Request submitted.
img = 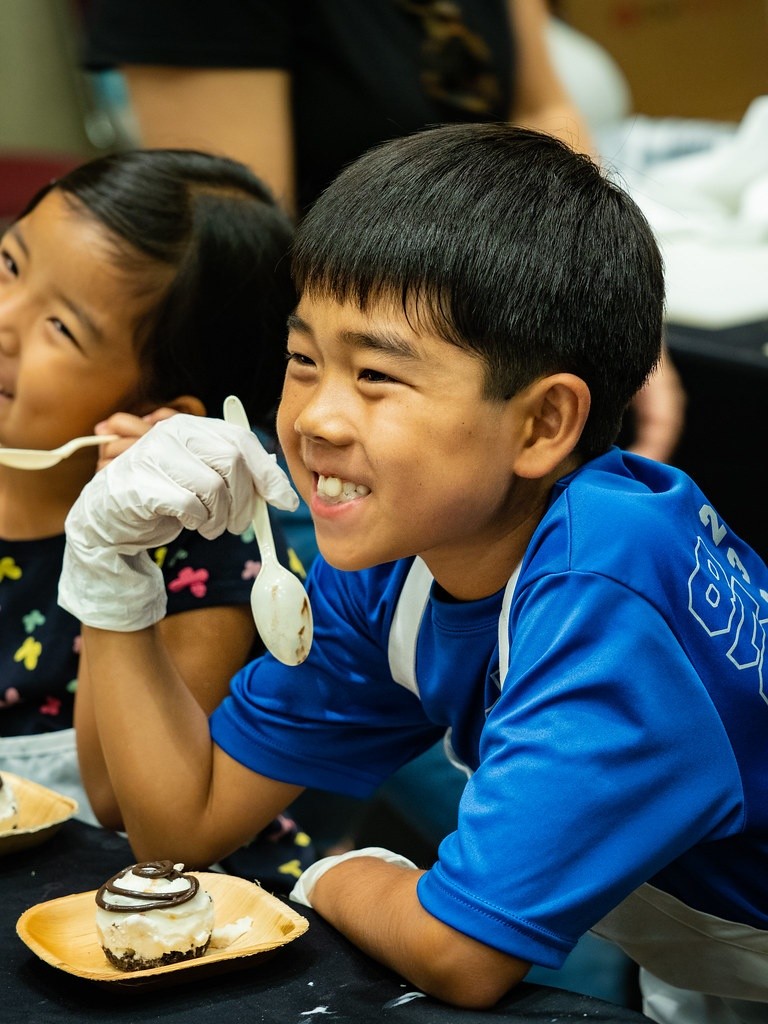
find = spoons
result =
[0,434,119,471]
[223,396,314,667]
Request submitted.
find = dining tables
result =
[0,819,658,1024]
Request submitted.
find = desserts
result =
[0,774,19,832]
[94,858,214,972]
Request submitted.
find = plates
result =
[0,770,79,851]
[16,872,311,981]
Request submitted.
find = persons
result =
[62,1,685,459]
[1,148,297,831]
[58,126,768,1006]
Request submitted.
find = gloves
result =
[287,847,419,908]
[58,413,304,632]
